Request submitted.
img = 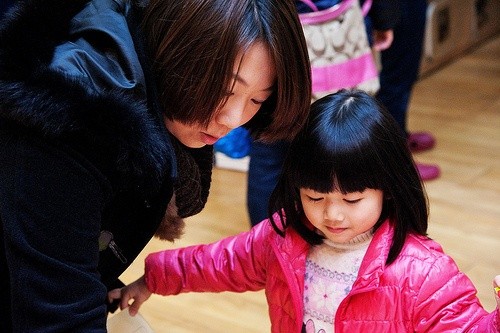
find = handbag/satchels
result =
[298,2,380,101]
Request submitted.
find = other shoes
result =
[405,131,435,150]
[414,160,439,181]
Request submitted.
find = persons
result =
[246,0,394,227]
[375,0,441,182]
[108,87,500,333]
[0,1,312,332]
